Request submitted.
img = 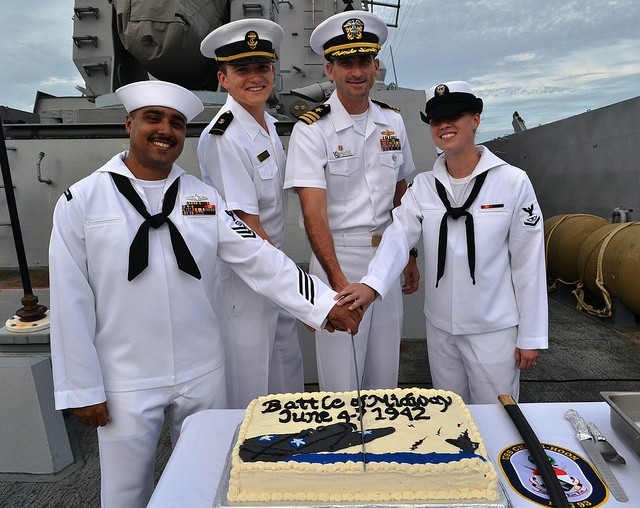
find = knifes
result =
[347,325,366,472]
[563,408,631,503]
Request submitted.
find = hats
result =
[114,80,204,124]
[199,18,285,66]
[420,80,483,125]
[309,9,388,59]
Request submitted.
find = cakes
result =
[225,386,502,503]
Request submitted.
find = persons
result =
[47,80,362,507]
[333,82,550,403]
[198,18,306,409]
[281,10,420,390]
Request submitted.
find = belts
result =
[334,235,383,247]
